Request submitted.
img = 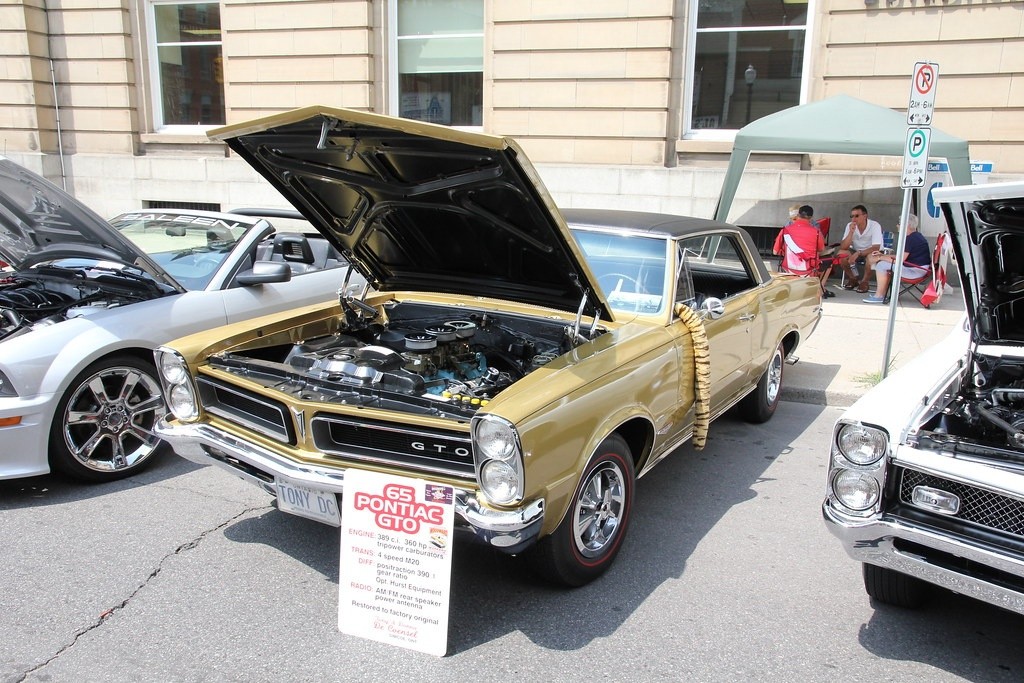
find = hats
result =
[799,205,813,219]
[787,204,801,219]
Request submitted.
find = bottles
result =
[849,263,858,277]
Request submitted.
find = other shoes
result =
[823,290,835,299]
[856,282,869,293]
[845,278,859,289]
[863,295,890,303]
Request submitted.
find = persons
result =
[862,213,932,303]
[840,205,885,294]
[785,205,835,298]
[773,205,824,278]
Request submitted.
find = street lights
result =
[744,65,756,125]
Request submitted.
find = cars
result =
[823,181,1024,618]
[151,105,824,590]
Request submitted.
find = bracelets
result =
[859,250,862,254]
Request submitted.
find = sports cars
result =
[0,155,376,483]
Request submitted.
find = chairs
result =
[883,234,944,309]
[817,218,830,246]
[206,228,322,277]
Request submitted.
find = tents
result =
[698,92,972,265]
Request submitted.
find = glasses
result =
[850,214,865,218]
